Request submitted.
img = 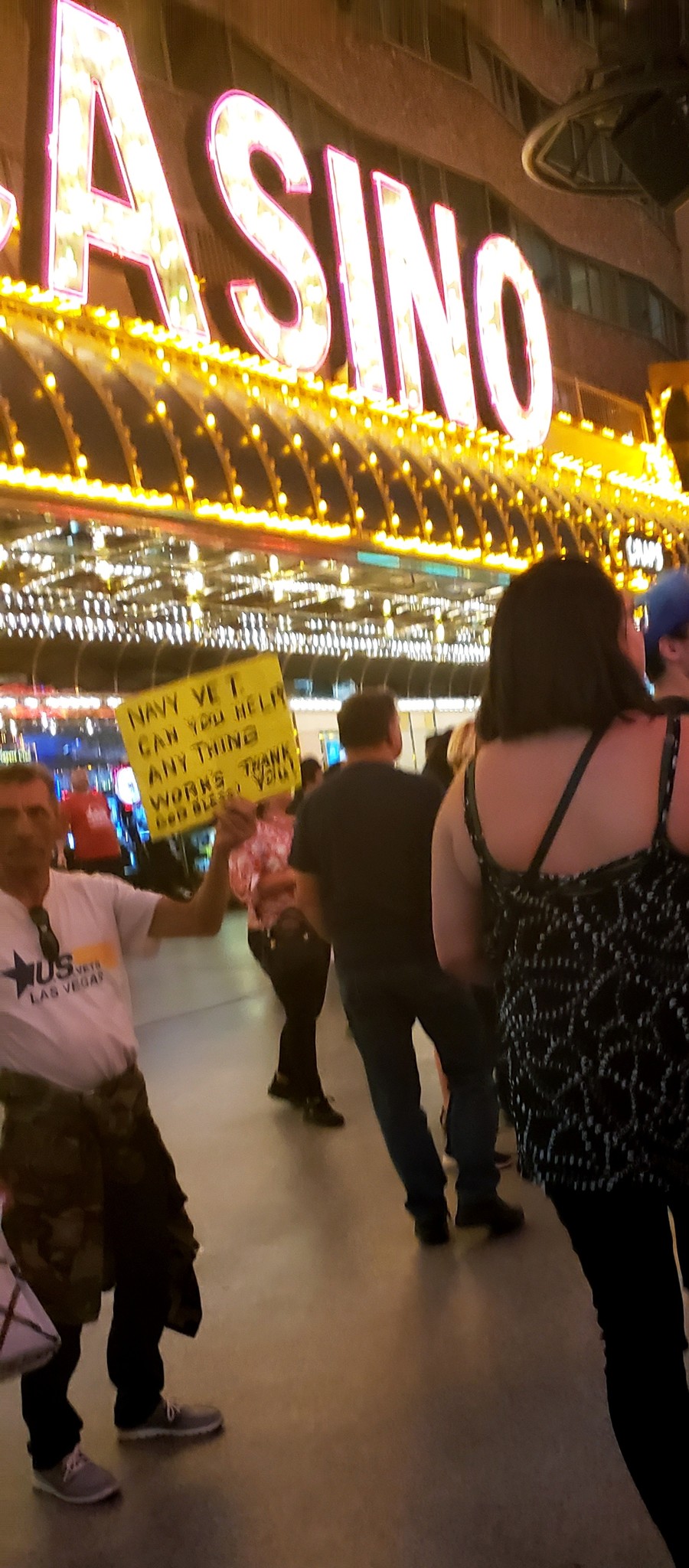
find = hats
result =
[631,575,689,641]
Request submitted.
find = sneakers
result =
[116,1396,222,1439]
[32,1448,118,1505]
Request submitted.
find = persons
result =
[54,765,127,879]
[430,558,689,1568]
[0,765,256,1504]
[286,692,522,1243]
[228,758,346,1126]
[643,566,689,700]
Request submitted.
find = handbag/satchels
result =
[249,849,318,961]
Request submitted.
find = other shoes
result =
[413,1217,449,1246]
[267,1073,300,1102]
[457,1197,525,1228]
[305,1101,347,1127]
